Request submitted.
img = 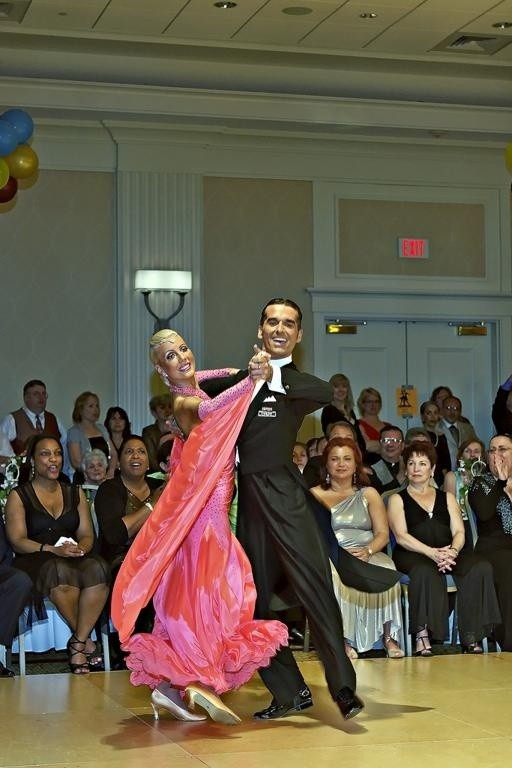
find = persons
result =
[165,298,365,724]
[110,329,290,724]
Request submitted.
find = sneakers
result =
[0,660,16,678]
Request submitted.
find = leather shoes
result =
[252,686,314,723]
[331,690,365,722]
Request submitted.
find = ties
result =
[33,415,44,434]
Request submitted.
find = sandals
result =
[66,632,104,675]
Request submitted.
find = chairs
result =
[14,483,111,677]
[380,487,488,658]
[464,491,504,653]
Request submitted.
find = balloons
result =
[0,108,39,215]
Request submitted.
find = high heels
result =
[381,634,405,659]
[460,640,483,655]
[413,634,434,656]
[345,647,359,659]
[148,687,207,722]
[184,681,242,727]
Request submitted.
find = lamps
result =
[133,269,192,331]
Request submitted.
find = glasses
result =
[442,404,458,414]
[378,437,401,446]
[486,445,509,456]
[462,446,483,457]
